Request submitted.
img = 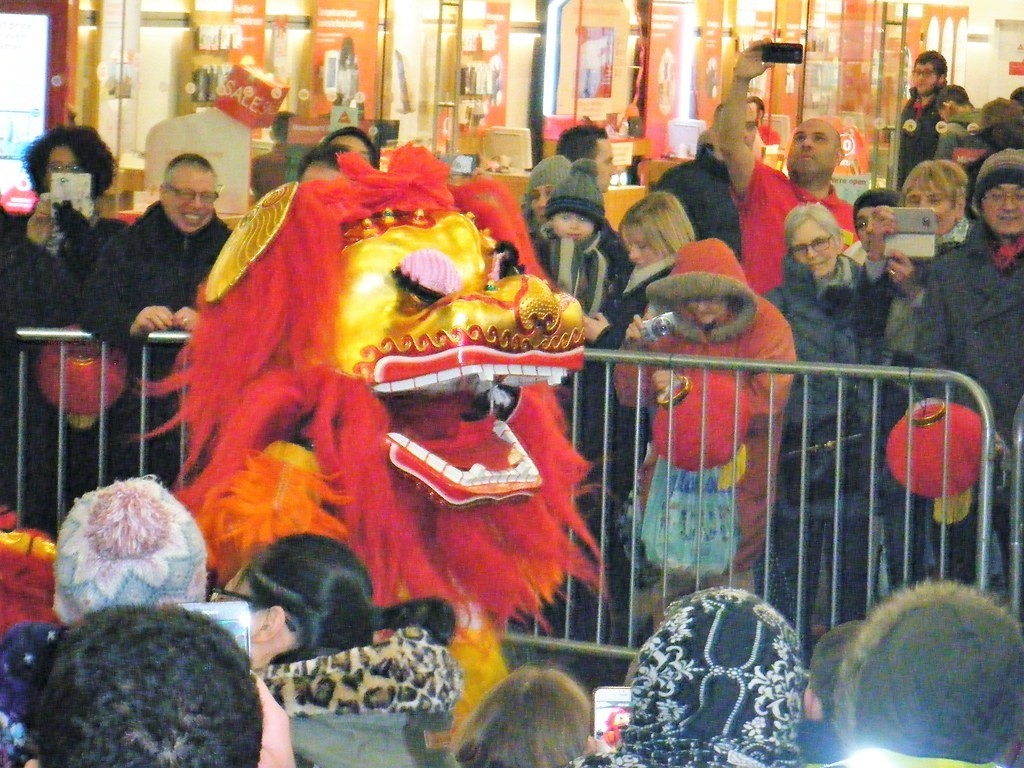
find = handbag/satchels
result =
[641,451,738,578]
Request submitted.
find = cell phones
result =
[888,207,935,234]
[49,173,92,219]
[181,600,252,659]
[590,686,634,755]
[762,42,803,64]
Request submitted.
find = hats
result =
[974,149,1024,203]
[51,481,209,628]
[546,156,606,224]
[853,187,900,218]
[526,154,573,190]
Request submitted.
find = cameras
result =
[640,312,677,344]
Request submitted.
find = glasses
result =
[165,185,220,204]
[854,218,870,232]
[48,163,85,174]
[789,235,836,257]
[203,583,296,640]
[910,68,938,78]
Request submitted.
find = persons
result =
[0,35,1023,768]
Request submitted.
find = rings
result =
[887,269,896,277]
[181,317,189,325]
